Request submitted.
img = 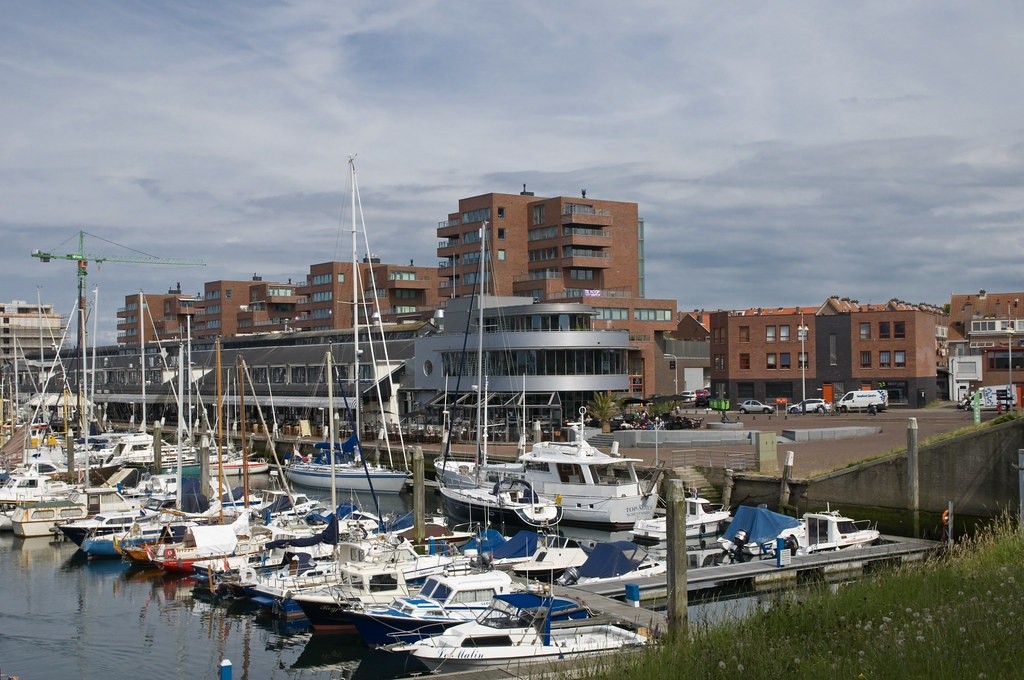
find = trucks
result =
[957,384,1018,412]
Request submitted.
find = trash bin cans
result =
[709,398,730,410]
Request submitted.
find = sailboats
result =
[1,156,881,676]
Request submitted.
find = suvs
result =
[787,398,830,415]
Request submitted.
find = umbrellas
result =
[399,411,432,428]
[619,397,649,414]
[364,409,392,424]
[653,395,687,401]
[25,395,95,407]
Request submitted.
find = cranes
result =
[30,231,207,359]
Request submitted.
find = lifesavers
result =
[240,568,257,583]
[163,548,176,559]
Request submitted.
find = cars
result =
[695,389,710,398]
[738,399,776,415]
[679,390,698,403]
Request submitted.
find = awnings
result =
[91,394,363,410]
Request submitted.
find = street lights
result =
[443,411,452,452]
[213,403,218,435]
[798,312,809,415]
[1003,314,1016,413]
[129,401,134,429]
[318,407,325,433]
[655,417,659,467]
[663,354,678,394]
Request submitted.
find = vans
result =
[835,389,889,413]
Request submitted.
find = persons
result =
[634,409,648,420]
